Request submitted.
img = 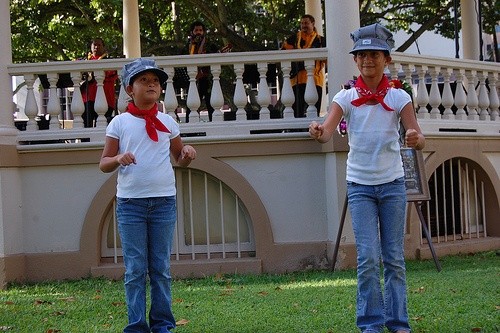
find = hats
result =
[122,58,168,89]
[349,23,395,54]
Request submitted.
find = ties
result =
[127,101,171,142]
[351,75,395,111]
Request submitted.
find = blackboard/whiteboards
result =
[400,149,423,196]
